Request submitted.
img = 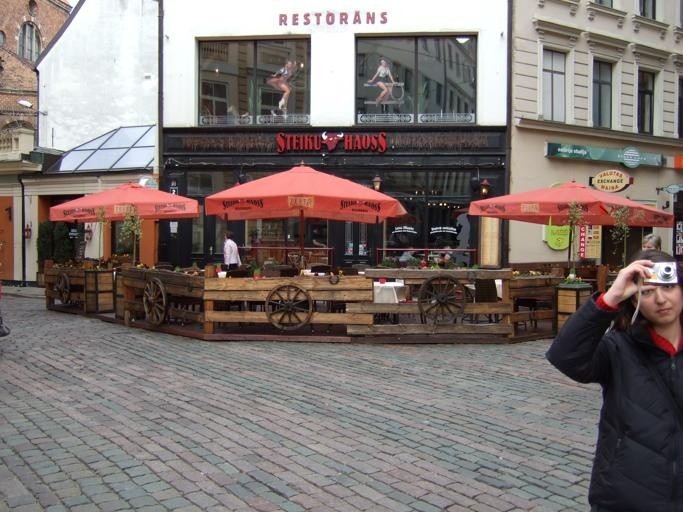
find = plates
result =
[302,270,325,277]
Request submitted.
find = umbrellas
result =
[468,179,674,270]
[48,181,198,266]
[204,160,406,269]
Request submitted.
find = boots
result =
[279,98,285,110]
[375,97,383,109]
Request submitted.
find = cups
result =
[218,271,226,278]
[357,272,365,276]
[379,279,386,284]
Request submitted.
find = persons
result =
[640,232,663,254]
[222,228,243,278]
[398,247,417,261]
[545,249,683,511]
[366,58,398,111]
[308,229,326,263]
[267,59,297,115]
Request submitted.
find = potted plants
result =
[569,268,577,281]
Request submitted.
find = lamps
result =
[17,100,47,116]
[84,224,92,240]
[371,174,383,190]
[24,224,32,239]
[479,179,491,199]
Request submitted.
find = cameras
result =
[642,261,678,285]
